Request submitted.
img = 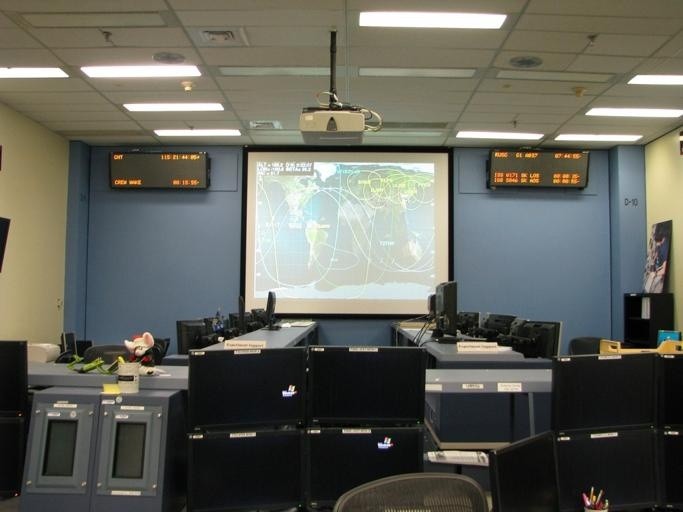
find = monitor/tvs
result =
[307,426,422,512]
[205,318,239,342]
[186,430,307,511]
[490,431,558,512]
[84,344,132,362]
[659,429,682,511]
[512,320,562,358]
[266,291,280,333]
[0,415,30,503]
[457,311,479,337]
[477,312,517,342]
[431,281,459,344]
[556,429,662,511]
[552,353,658,433]
[657,354,683,433]
[251,308,267,329]
[310,345,426,428]
[242,312,259,334]
[229,313,245,336]
[0,340,28,416]
[176,319,218,355]
[189,348,309,432]
[60,332,78,357]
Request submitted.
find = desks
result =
[203,320,317,350]
[388,320,525,365]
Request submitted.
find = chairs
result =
[568,337,612,357]
[334,471,488,511]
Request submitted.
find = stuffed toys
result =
[124,332,157,376]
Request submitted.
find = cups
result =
[583,506,609,512]
[118,363,140,393]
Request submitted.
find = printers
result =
[28,343,61,364]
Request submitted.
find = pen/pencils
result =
[581,486,609,510]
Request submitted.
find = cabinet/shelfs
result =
[623,292,674,348]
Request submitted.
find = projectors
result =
[299,105,364,146]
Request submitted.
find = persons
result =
[640,223,670,293]
